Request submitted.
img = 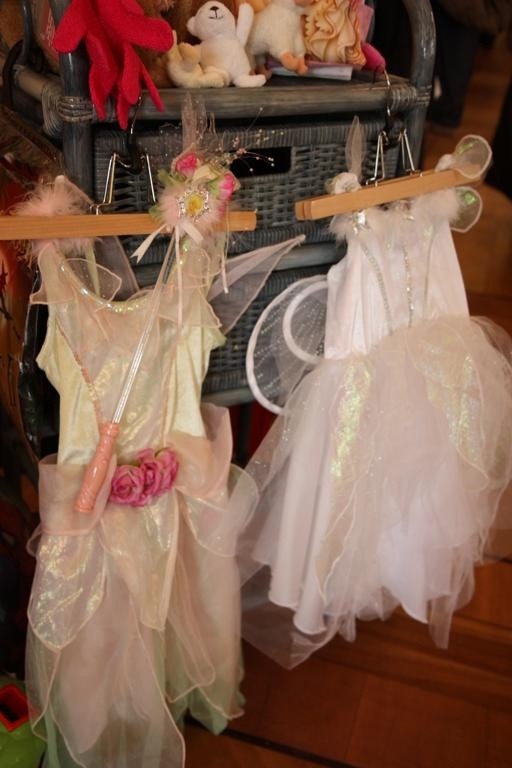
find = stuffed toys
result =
[242,1,308,80]
[161,31,231,90]
[185,1,265,89]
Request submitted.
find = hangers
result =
[1,95,257,243]
[294,63,481,222]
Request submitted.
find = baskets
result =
[95,120,403,267]
[134,243,347,392]
